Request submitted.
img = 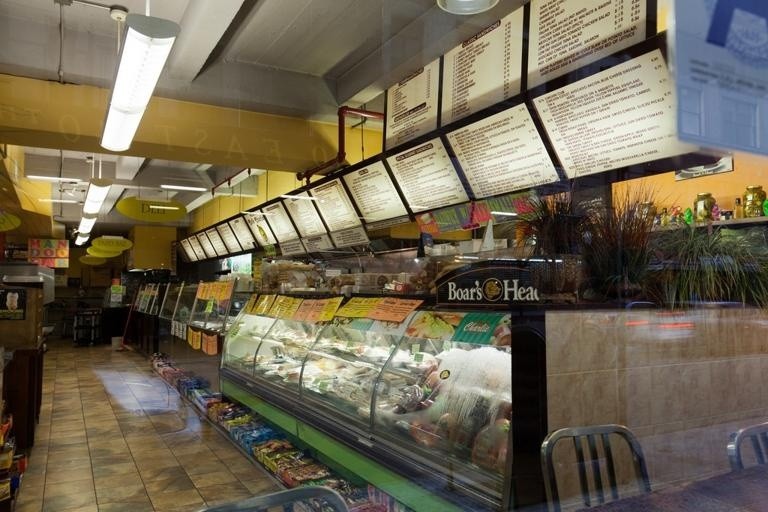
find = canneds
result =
[639,202,658,226]
[693,192,717,221]
[719,209,733,221]
[742,185,766,216]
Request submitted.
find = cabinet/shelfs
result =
[0,275,46,485]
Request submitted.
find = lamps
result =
[57,0,181,246]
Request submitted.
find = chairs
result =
[539,422,766,511]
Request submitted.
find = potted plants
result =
[500,172,768,310]
